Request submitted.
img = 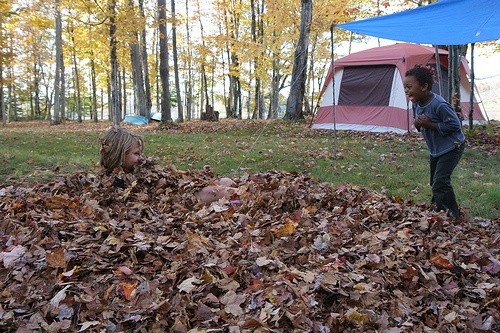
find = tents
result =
[305,42,491,136]
[331,0,500,229]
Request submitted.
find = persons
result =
[403,66,468,221]
[97,125,249,203]
